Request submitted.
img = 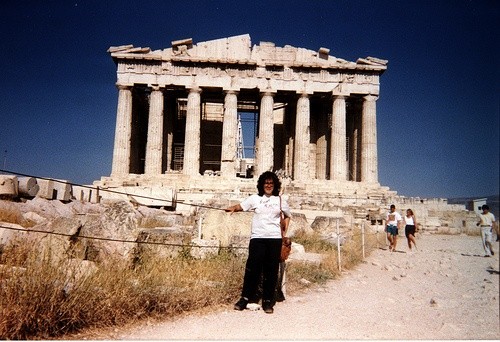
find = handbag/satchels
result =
[280,237,292,263]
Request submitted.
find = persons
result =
[385,205,403,252]
[401,209,419,252]
[225,171,291,314]
[476,205,496,257]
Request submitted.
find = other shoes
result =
[262,301,273,314]
[235,297,249,310]
